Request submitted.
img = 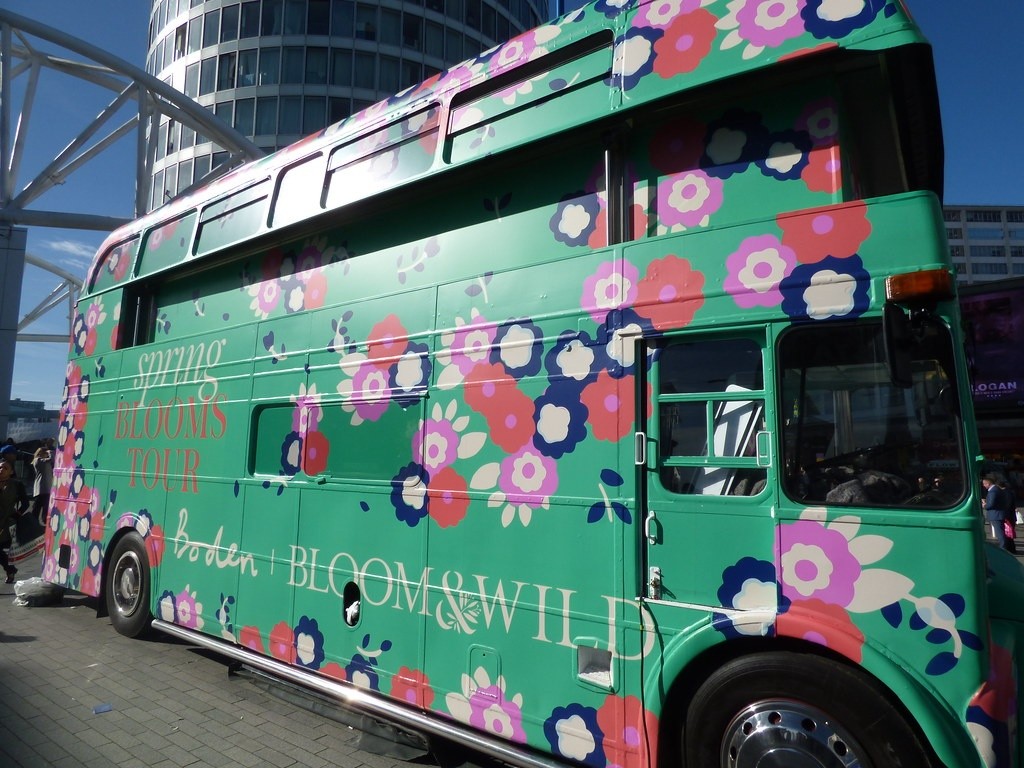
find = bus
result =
[42,0,1023,768]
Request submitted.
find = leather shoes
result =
[5,569,17,582]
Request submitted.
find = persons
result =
[982,477,1011,549]
[997,479,1017,553]
[0,459,29,584]
[2,438,17,479]
[29,447,53,525]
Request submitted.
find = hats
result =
[0,445,21,455]
